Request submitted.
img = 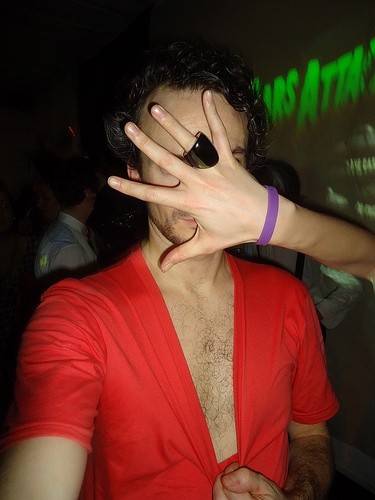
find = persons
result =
[0,41,341,500]
[238,160,364,345]
[107,90,375,285]
[34,157,100,294]
[0,172,72,400]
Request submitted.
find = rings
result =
[183,131,219,169]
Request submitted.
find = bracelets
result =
[256,184,279,246]
